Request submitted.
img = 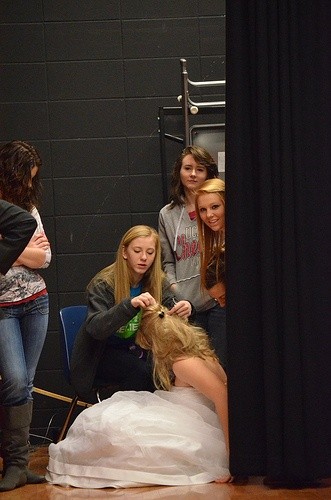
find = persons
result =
[157,144,225,373]
[68,224,196,404]
[0,139,52,492]
[203,250,225,310]
[195,179,225,283]
[0,198,38,276]
[47,304,229,489]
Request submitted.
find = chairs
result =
[58,305,89,442]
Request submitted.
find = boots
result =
[25,400,46,483]
[0,401,33,493]
